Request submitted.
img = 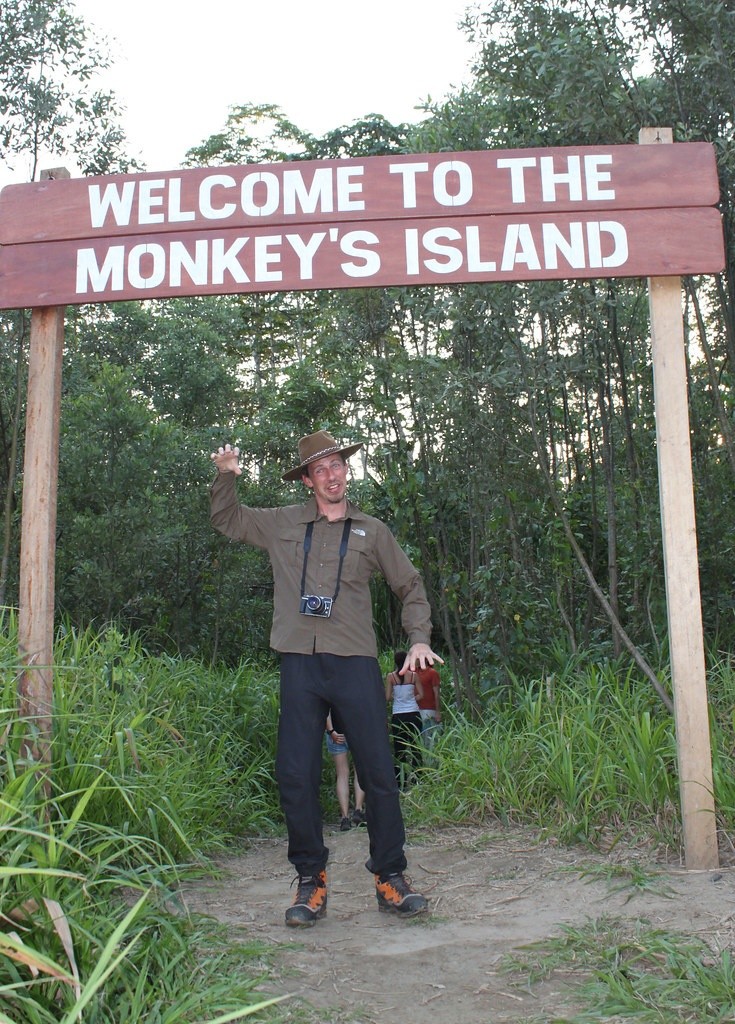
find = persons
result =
[208,429,445,926]
[325,651,441,830]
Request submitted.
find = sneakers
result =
[340,818,352,831]
[284,869,328,926]
[353,809,366,825]
[374,873,429,919]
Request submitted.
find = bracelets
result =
[327,729,335,735]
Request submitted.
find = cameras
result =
[300,595,333,619]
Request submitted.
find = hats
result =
[281,428,364,481]
